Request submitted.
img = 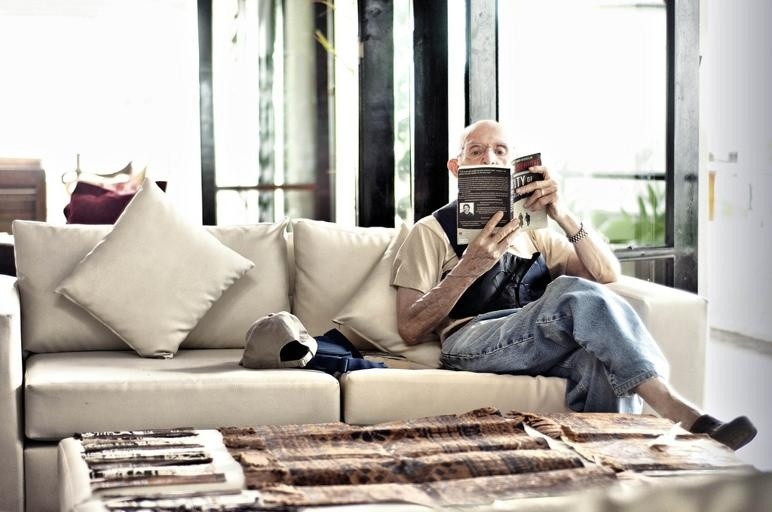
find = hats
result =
[241,311,317,368]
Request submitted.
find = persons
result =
[389,120,758,451]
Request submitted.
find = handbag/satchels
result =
[311,329,387,372]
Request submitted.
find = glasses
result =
[456,145,510,159]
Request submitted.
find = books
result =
[457,153,547,245]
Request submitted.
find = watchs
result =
[565,223,588,243]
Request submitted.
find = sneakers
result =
[690,414,756,451]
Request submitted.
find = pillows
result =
[64,180,168,226]
[330,218,444,370]
[290,215,401,354]
[11,216,294,360]
[53,176,257,361]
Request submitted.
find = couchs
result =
[0,218,708,512]
[56,412,745,511]
